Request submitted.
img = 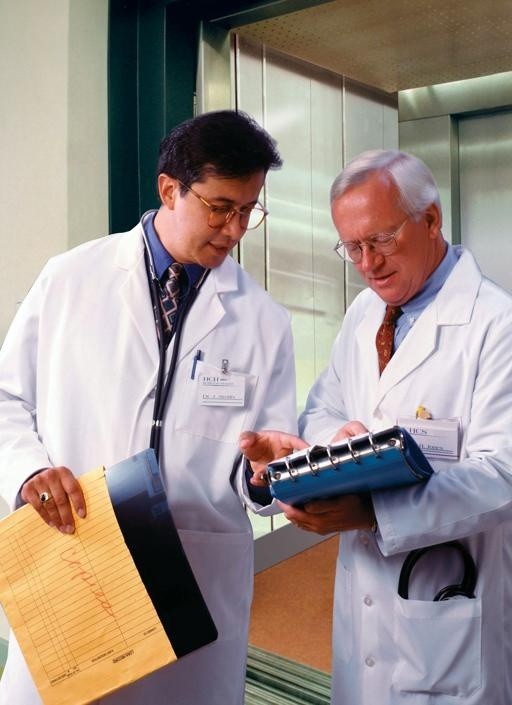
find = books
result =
[265,427,432,506]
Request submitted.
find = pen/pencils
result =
[190,350,202,381]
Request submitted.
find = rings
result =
[39,492,52,502]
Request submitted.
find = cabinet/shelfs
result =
[227,33,400,575]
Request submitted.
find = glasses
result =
[332,211,412,262]
[172,177,269,232]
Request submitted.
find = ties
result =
[153,262,183,354]
[375,304,404,377]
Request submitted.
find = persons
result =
[3,109,312,703]
[299,149,512,704]
